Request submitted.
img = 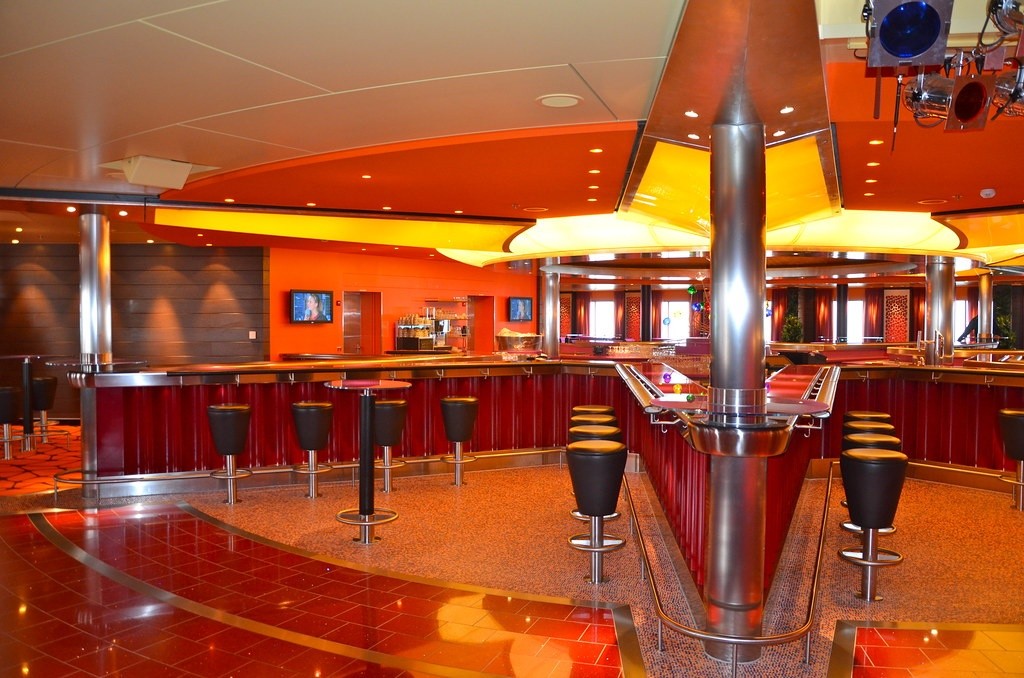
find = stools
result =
[30,377,57,443]
[292,401,334,498]
[441,397,478,487]
[209,404,251,504]
[373,400,407,492]
[997,409,1024,511]
[0,387,26,460]
[839,412,909,602]
[566,405,628,588]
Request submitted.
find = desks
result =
[0,354,71,451]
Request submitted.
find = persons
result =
[956,315,977,341]
[516,300,528,318]
[304,293,328,321]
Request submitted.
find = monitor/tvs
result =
[290,289,333,324]
[508,297,532,321]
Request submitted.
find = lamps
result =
[864,0,1024,131]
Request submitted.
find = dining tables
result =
[323,381,412,546]
